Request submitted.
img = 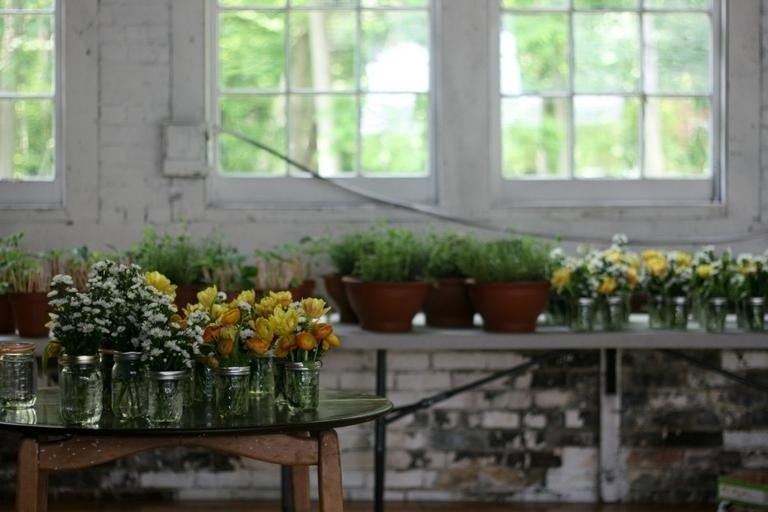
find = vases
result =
[0,342,39,424]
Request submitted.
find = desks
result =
[1,323,768,512]
[0,375,394,512]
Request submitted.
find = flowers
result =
[549,231,768,331]
